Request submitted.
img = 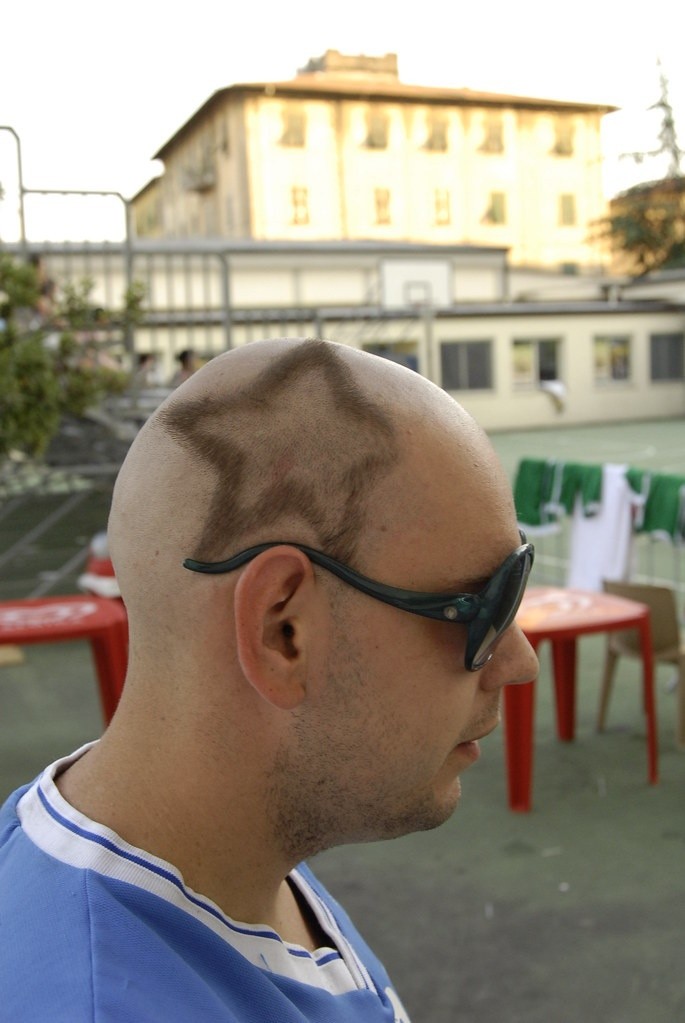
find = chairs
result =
[598,581,685,745]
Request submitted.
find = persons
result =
[0,337,539,1023]
[130,348,198,389]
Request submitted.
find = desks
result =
[480,584,661,814]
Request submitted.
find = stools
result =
[0,595,129,728]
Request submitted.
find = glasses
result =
[184,527,538,674]
